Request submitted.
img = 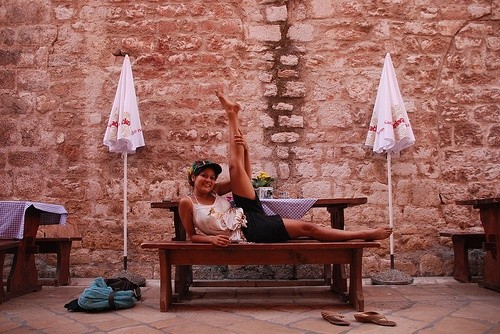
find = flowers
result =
[252,172,274,188]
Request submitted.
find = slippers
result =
[321,311,350,324]
[354,312,396,326]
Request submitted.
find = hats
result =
[190,160,222,175]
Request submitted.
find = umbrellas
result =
[103,53,146,271]
[366,51,415,270]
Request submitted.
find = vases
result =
[255,187,273,199]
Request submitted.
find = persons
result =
[178,88,393,247]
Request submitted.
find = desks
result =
[456,197,500,293]
[151,197,368,299]
[0,201,67,297]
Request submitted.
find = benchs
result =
[141,237,380,313]
[439,231,500,293]
[0,237,82,304]
